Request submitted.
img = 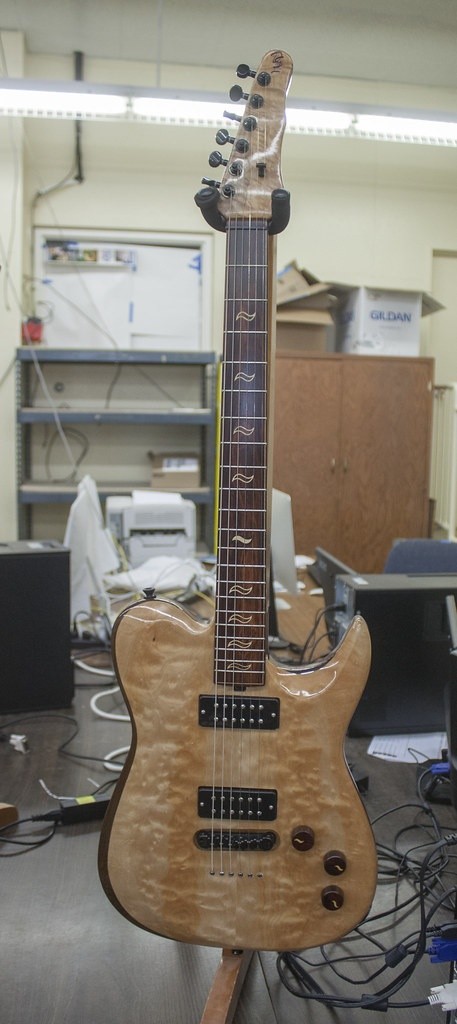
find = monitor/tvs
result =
[316,547,360,648]
[270,486,298,648]
[417,595,456,805]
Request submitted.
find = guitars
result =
[93,48,376,951]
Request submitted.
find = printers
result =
[105,497,197,571]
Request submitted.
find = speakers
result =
[0,539,75,711]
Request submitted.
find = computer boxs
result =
[333,572,457,737]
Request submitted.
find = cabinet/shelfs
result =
[273,350,435,573]
[18,345,218,565]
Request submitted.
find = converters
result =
[32,794,112,824]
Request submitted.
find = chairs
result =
[384,537,457,574]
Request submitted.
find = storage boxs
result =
[148,450,204,487]
[328,281,447,356]
[275,307,334,350]
